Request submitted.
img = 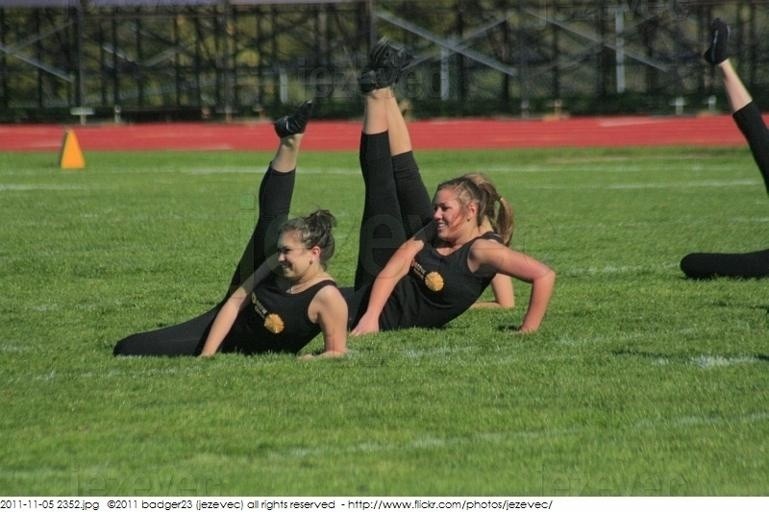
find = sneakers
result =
[275,97,316,139]
[357,36,402,92]
[703,17,731,65]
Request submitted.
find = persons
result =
[681,18,768,277]
[333,50,556,337]
[367,35,517,310]
[113,99,348,358]
